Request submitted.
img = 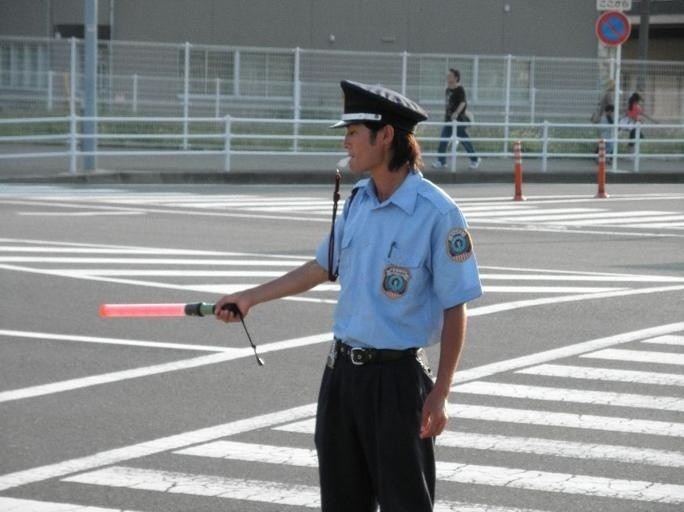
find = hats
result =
[328,80,427,133]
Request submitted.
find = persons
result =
[602,79,615,119]
[214,81,484,512]
[430,69,483,170]
[626,92,658,147]
[596,104,616,165]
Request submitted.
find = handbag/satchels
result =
[464,110,473,121]
[619,117,634,131]
[590,106,601,124]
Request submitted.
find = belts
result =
[334,341,418,362]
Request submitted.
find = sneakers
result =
[469,158,481,168]
[431,161,448,168]
